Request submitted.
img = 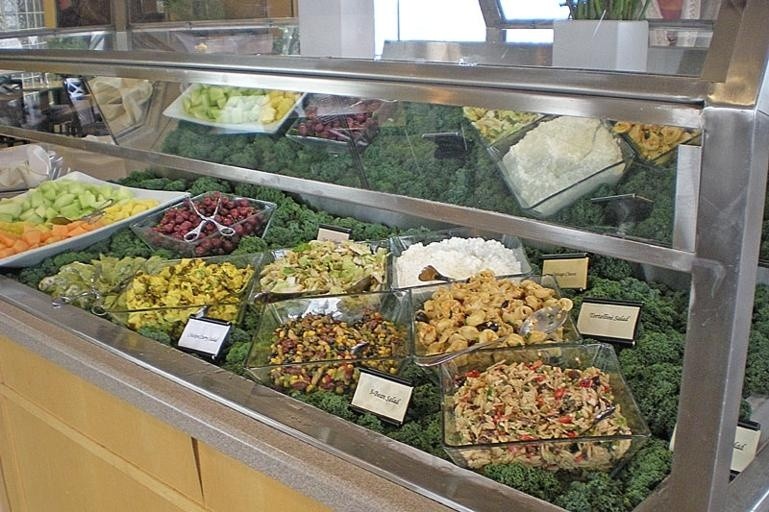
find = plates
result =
[1,171,193,268]
[164,83,312,133]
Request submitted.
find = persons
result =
[647,21,715,49]
[633,0,720,21]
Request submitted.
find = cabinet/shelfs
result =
[0,0,769,512]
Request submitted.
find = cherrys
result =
[146,192,268,257]
[299,98,369,142]
